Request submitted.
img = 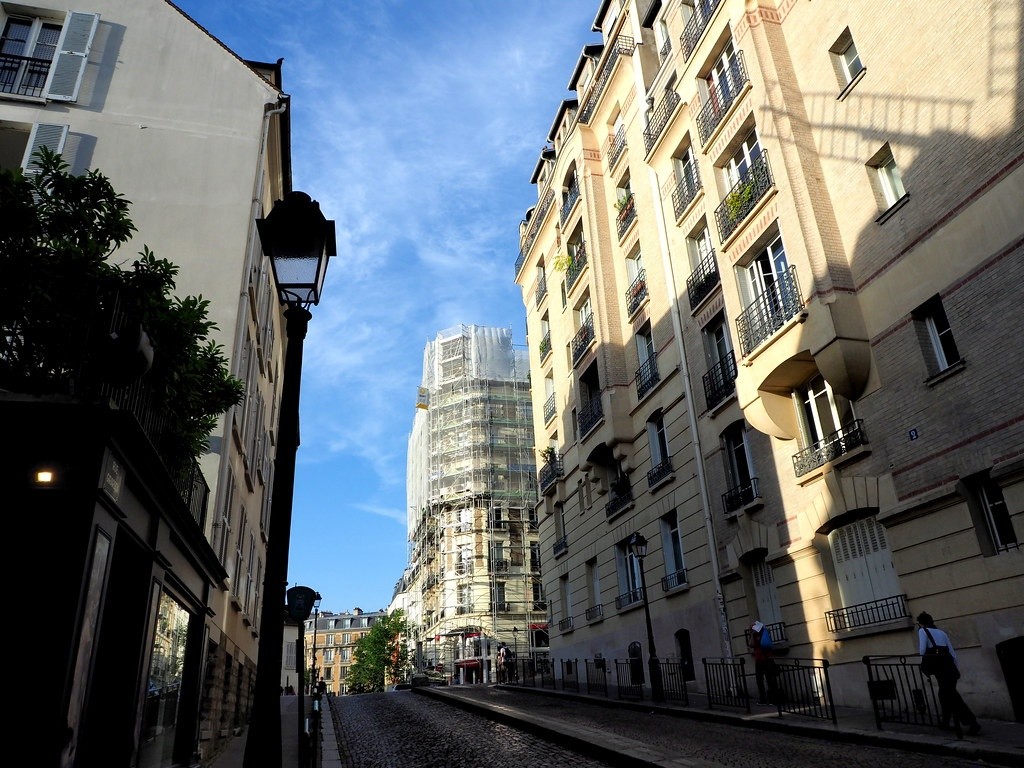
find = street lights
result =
[310,591,323,696]
[511,626,518,678]
[629,531,666,704]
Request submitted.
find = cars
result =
[391,682,412,692]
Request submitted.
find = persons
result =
[318,676,327,693]
[748,622,778,707]
[287,685,296,695]
[916,611,982,736]
[496,642,518,683]
[393,673,401,691]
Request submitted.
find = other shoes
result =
[756,701,767,706]
[769,701,773,705]
[939,723,950,733]
[965,723,981,736]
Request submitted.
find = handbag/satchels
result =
[921,628,950,673]
[761,628,774,654]
